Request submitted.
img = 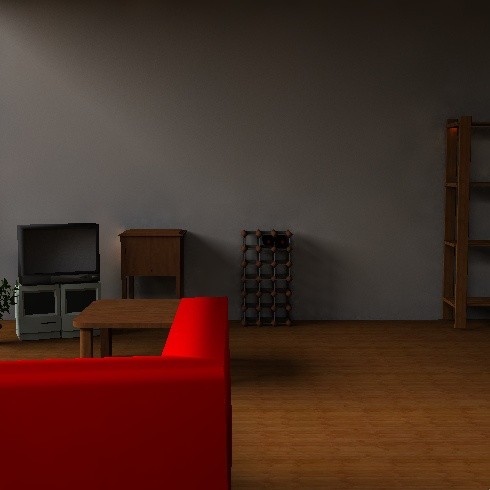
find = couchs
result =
[0,296,231,490]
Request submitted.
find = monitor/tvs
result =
[17,222,101,285]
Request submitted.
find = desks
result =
[118,229,187,298]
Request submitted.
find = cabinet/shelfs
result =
[15,283,101,341]
[239,229,292,326]
[442,115,490,329]
